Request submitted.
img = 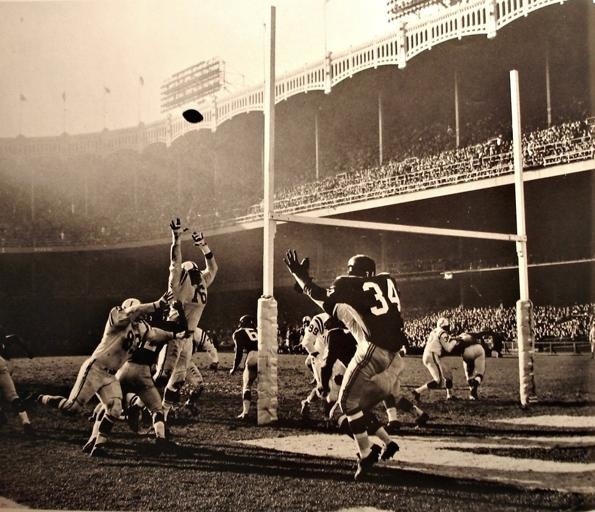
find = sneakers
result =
[411,390,420,401]
[24,424,33,434]
[16,392,39,412]
[157,445,182,457]
[354,444,381,481]
[83,409,125,458]
[128,405,201,432]
[382,442,398,460]
[447,396,460,402]
[468,379,478,399]
[294,257,309,294]
[299,400,428,438]
[236,414,248,420]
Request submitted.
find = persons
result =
[411,317,486,399]
[206,246,595,353]
[1,356,34,432]
[284,248,404,479]
[301,311,428,431]
[127,218,218,435]
[0,90,594,245]
[21,291,188,456]
[230,315,258,418]
[81,301,219,451]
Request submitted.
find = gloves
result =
[174,330,194,339]
[192,231,208,251]
[283,249,307,280]
[170,218,188,244]
[154,291,174,309]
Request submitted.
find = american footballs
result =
[182,109,203,123]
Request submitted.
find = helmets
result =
[346,255,375,277]
[121,298,163,321]
[238,314,257,328]
[437,318,450,331]
[181,260,200,284]
